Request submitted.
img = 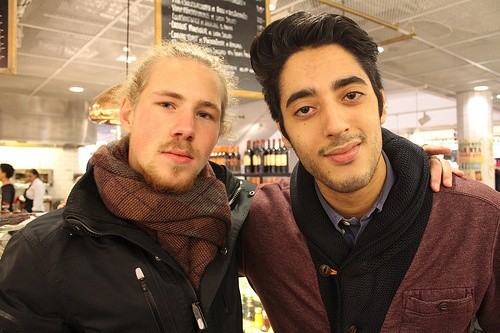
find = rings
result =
[427,155,440,161]
[420,144,428,147]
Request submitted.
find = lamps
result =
[88,0,131,125]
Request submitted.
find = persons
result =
[0,40,465,333]
[231,11,500,333]
[0,163,15,213]
[23,169,45,213]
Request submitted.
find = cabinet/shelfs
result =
[229,172,294,333]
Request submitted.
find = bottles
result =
[276,139,281,174]
[252,140,260,173]
[209,145,241,173]
[266,140,271,173]
[241,294,270,332]
[281,147,288,174]
[245,141,252,173]
[260,139,266,173]
[253,141,255,150]
[271,139,276,173]
